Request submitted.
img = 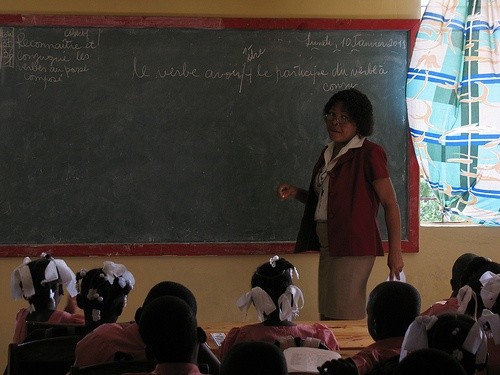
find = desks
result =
[196,311,375,362]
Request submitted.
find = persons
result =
[278,88,404,321]
[4,254,500,375]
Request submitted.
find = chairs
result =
[7,333,84,375]
[23,319,85,335]
[70,357,210,375]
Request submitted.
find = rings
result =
[399,269,402,272]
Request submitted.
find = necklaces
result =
[317,168,331,186]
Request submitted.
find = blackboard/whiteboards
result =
[0,12,421,257]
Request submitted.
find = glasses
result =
[325,113,352,123]
